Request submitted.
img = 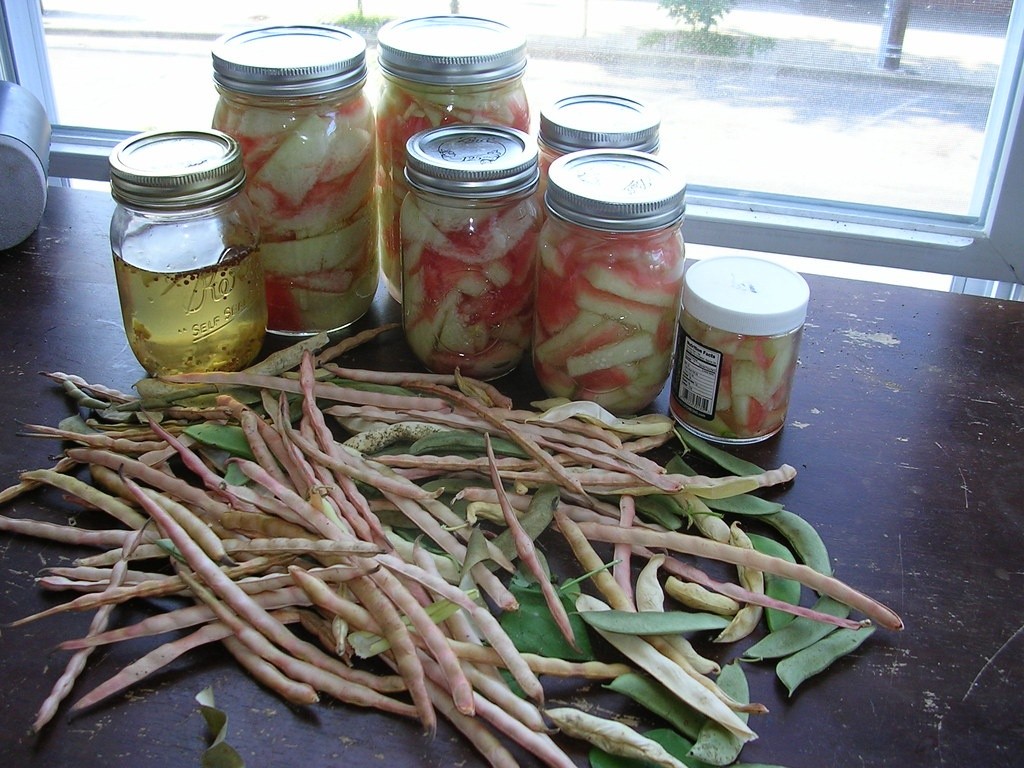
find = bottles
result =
[108,128,268,378]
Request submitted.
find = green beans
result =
[0,319,904,768]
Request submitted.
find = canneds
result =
[671,256,811,443]
